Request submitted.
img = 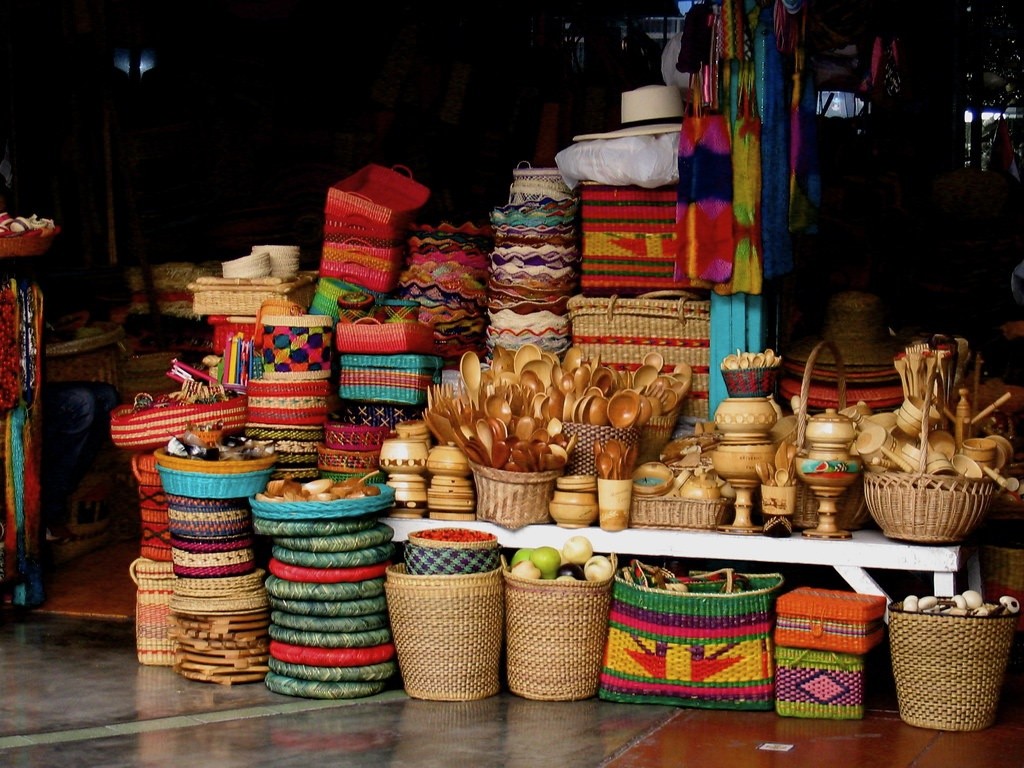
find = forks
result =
[427,384,465,457]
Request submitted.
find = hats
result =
[573,83,685,142]
[779,293,907,407]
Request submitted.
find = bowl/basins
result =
[549,491,598,528]
[714,398,777,437]
[0,213,33,234]
[302,479,332,501]
[380,439,428,477]
[427,442,471,480]
[806,409,854,448]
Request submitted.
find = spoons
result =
[595,439,632,480]
[544,386,652,429]
[755,442,797,487]
[466,394,578,473]
[592,352,692,418]
[460,351,481,411]
[721,349,782,370]
[492,344,592,394]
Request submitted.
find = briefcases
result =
[774,586,887,721]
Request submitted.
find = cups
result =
[598,477,632,531]
[761,485,796,515]
[855,396,1013,479]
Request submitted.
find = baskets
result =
[864,334,997,544]
[792,342,873,531]
[0,167,784,710]
[888,596,1018,732]
[136,664,605,768]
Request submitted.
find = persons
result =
[37,379,123,543]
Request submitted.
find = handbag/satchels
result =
[597,561,784,711]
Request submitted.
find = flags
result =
[991,118,1020,182]
